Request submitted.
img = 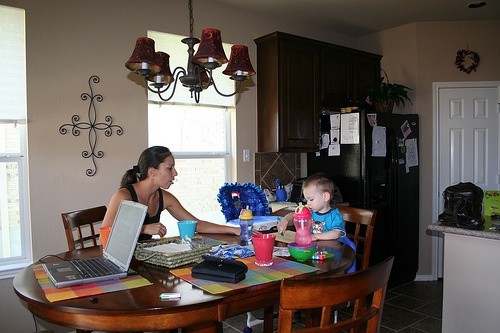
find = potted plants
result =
[359,77,413,114]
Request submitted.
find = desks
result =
[14,232,353,333]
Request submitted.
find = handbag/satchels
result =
[191,255,248,284]
[437,182,486,231]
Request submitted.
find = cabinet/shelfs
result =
[252,30,418,290]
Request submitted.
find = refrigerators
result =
[312,113,420,284]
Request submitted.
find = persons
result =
[99,146,262,241]
[277,173,357,273]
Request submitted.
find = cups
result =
[177,220,198,240]
[99,226,112,249]
[251,234,276,266]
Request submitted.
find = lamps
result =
[125,0,255,103]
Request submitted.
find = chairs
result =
[62,206,106,251]
[334,205,378,270]
[278,256,396,333]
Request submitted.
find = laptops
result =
[42,200,148,288]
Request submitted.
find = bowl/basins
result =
[287,241,318,262]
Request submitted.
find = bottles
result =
[239,205,254,242]
[293,202,313,246]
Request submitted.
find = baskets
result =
[133,238,212,268]
